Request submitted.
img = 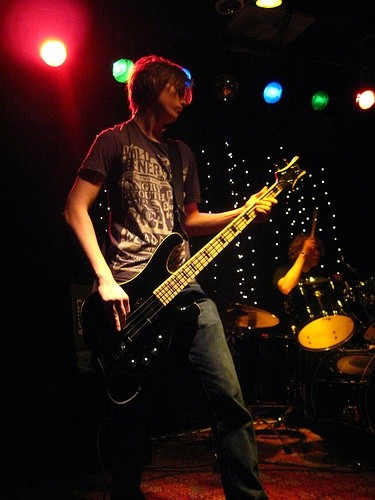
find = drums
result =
[310,349,375,454]
[351,278,375,342]
[284,277,355,351]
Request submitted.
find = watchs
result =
[301,252,308,256]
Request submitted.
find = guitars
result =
[79,156,307,404]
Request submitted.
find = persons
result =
[272,232,342,325]
[62,54,280,500]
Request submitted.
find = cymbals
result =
[222,304,279,328]
